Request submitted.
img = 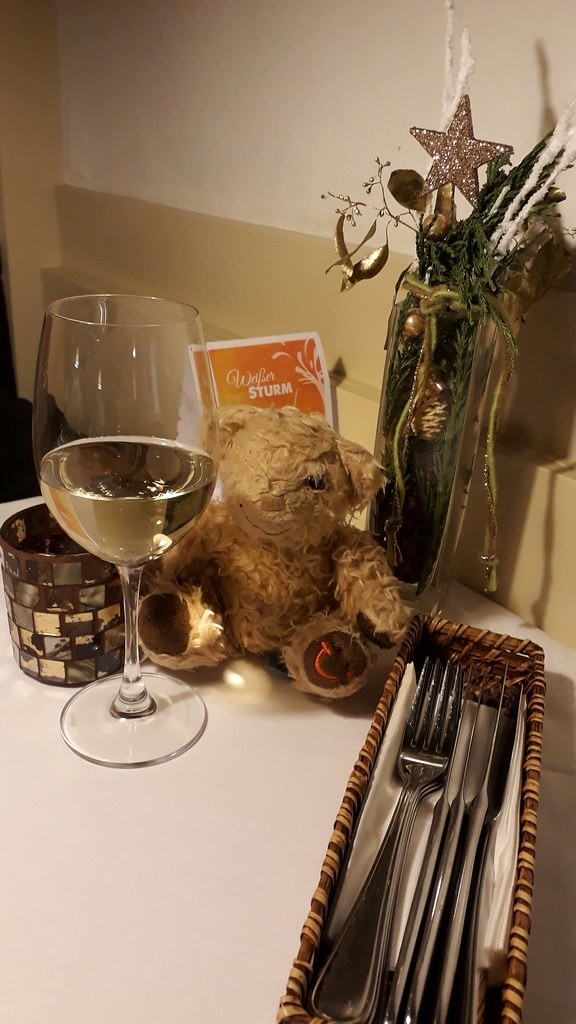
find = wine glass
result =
[32,291,224,769]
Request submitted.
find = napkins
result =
[323,662,526,990]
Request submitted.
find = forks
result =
[313,654,464,1024]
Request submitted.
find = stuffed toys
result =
[137,406,406,697]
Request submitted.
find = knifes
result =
[388,660,511,1024]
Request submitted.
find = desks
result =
[0,462,576,1024]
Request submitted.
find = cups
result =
[0,502,147,688]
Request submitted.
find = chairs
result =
[0,394,50,506]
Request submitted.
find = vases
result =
[364,271,504,603]
